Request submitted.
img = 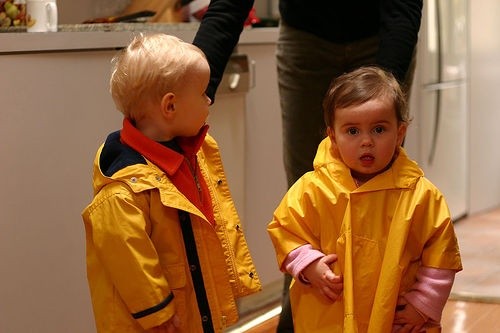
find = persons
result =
[266,67,463,333]
[193,0,423,333]
[81,35,262,333]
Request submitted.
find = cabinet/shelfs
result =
[0,26,286,333]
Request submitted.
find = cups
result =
[26,0,58,32]
